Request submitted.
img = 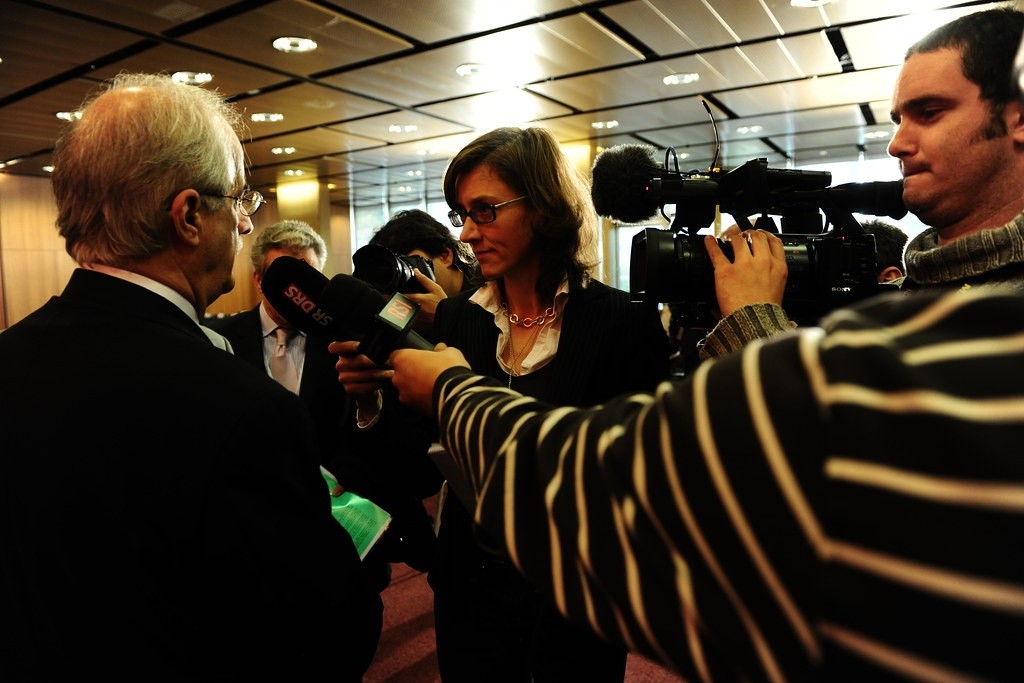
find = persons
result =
[205,7,1024,683]
[0,76,383,683]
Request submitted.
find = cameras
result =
[353,245,436,296]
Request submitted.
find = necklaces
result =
[499,296,554,328]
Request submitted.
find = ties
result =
[272,328,298,394]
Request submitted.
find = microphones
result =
[592,147,831,226]
[261,255,437,371]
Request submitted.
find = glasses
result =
[165,189,267,216]
[448,196,528,227]
